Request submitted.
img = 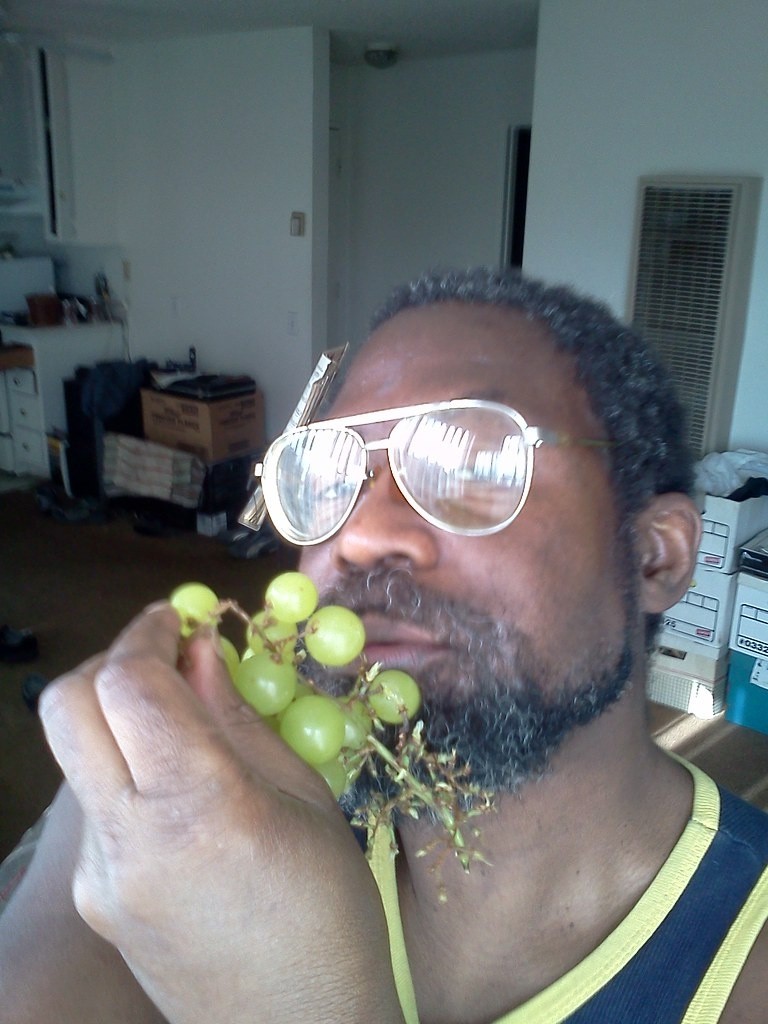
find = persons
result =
[0,268,768,1024]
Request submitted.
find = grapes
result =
[168,573,420,802]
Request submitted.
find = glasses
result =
[248,397,622,546]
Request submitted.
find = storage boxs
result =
[142,388,265,466]
[648,494,768,737]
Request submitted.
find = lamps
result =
[364,44,397,68]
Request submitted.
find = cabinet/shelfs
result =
[0,34,76,241]
[0,321,125,480]
